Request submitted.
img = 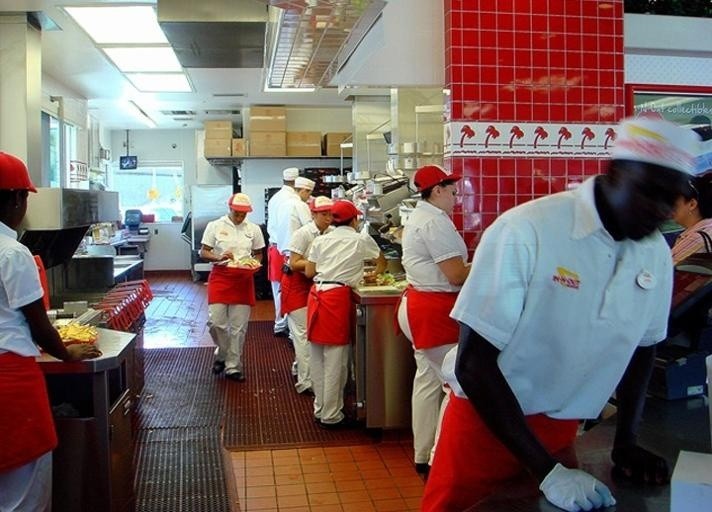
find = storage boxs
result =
[202,107,353,158]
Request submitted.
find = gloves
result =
[539,461,617,512]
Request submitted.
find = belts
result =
[314,281,346,286]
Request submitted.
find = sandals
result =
[212,359,224,374]
[225,372,246,383]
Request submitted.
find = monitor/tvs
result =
[120,156,137,169]
[125,209,143,230]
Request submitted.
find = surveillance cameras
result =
[172,144,176,148]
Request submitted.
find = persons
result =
[0,151,102,512]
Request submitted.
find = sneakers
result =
[415,462,431,478]
[314,414,357,430]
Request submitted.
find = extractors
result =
[0,10,121,272]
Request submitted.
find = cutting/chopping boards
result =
[356,282,398,292]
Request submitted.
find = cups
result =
[325,171,383,200]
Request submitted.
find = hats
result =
[309,195,334,212]
[228,192,254,213]
[330,200,364,223]
[604,116,704,177]
[414,166,461,192]
[294,176,317,191]
[0,149,39,195]
[282,167,299,182]
